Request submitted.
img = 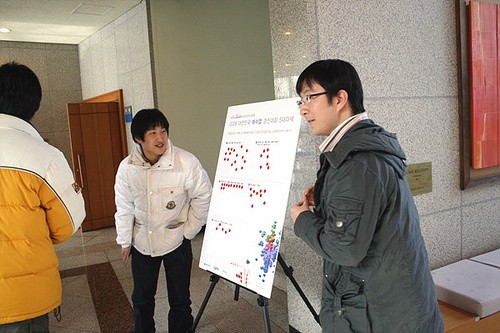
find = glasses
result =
[295,90,334,108]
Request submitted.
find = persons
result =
[1,61,86,332]
[289,60,445,333]
[115,108,213,333]
